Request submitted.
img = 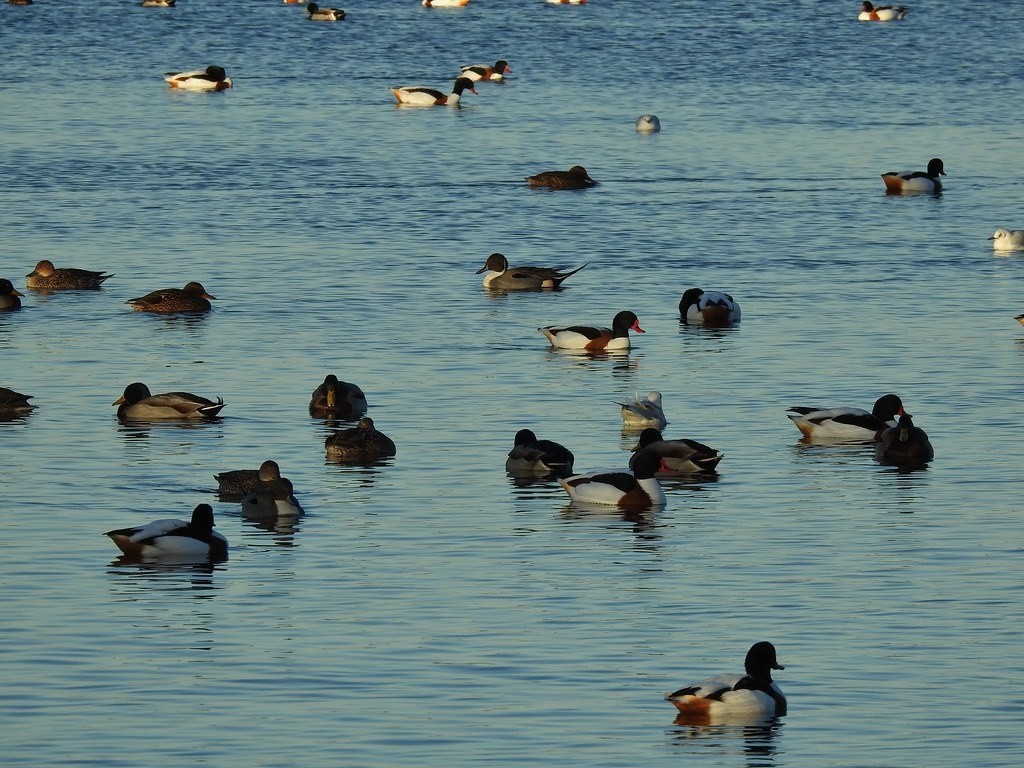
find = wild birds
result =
[505,310,726,510]
[283,0,588,21]
[662,642,789,719]
[457,60,512,81]
[142,0,233,92]
[0,259,396,568]
[523,165,598,192]
[857,2,1024,326]
[476,254,592,290]
[387,78,479,107]
[678,289,743,330]
[785,394,935,466]
[636,115,661,131]
